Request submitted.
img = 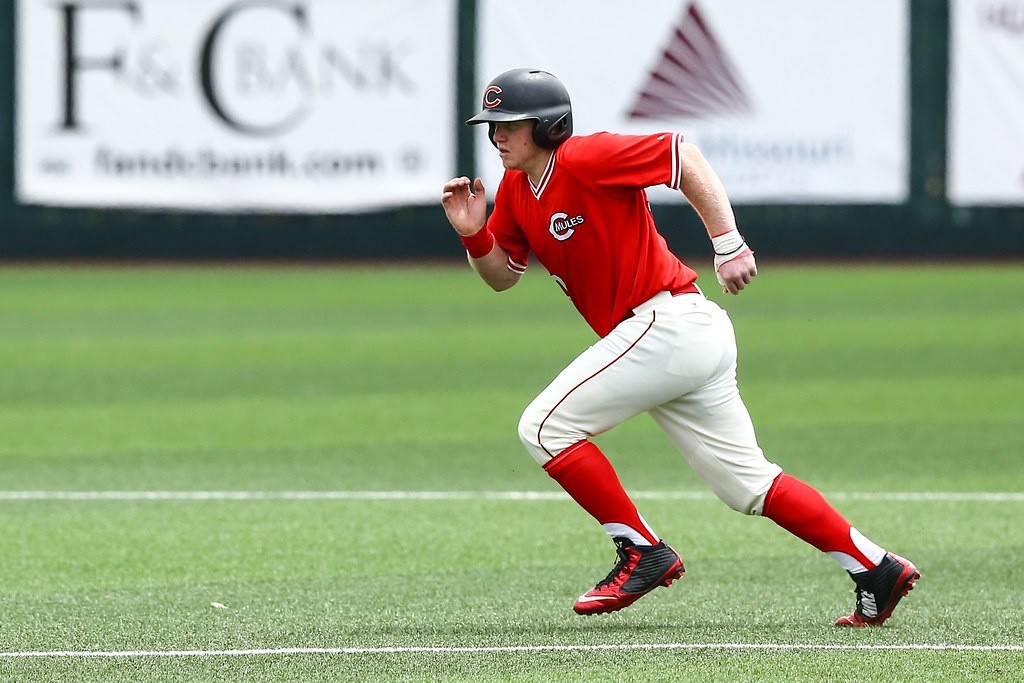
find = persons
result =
[439,68,921,629]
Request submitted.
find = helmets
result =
[465,68,574,150]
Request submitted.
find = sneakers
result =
[835,552,922,628]
[573,537,686,616]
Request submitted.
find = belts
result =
[620,284,700,320]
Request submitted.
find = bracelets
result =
[713,236,745,256]
[710,230,752,269]
[459,224,496,259]
